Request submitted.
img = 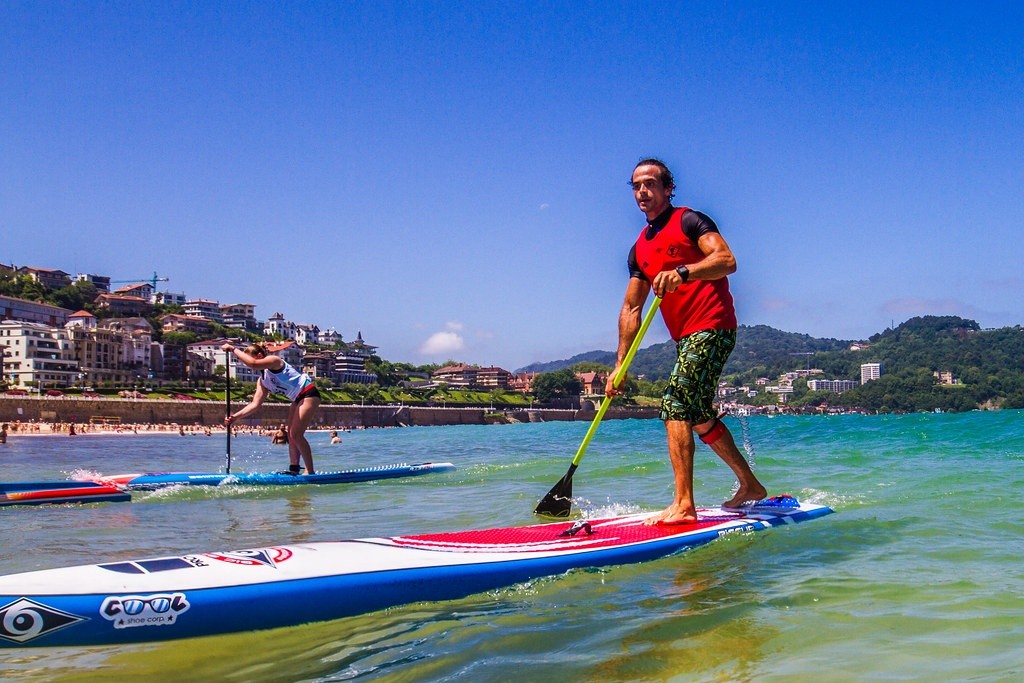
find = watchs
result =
[674,264,690,284]
[232,346,238,353]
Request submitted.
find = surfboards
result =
[0,467,456,505]
[0,496,835,649]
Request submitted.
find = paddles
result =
[224,349,231,473]
[533,292,661,518]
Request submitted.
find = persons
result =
[271,423,290,445]
[0,424,10,444]
[604,158,767,525]
[329,431,342,445]
[1,417,409,438]
[221,342,321,476]
